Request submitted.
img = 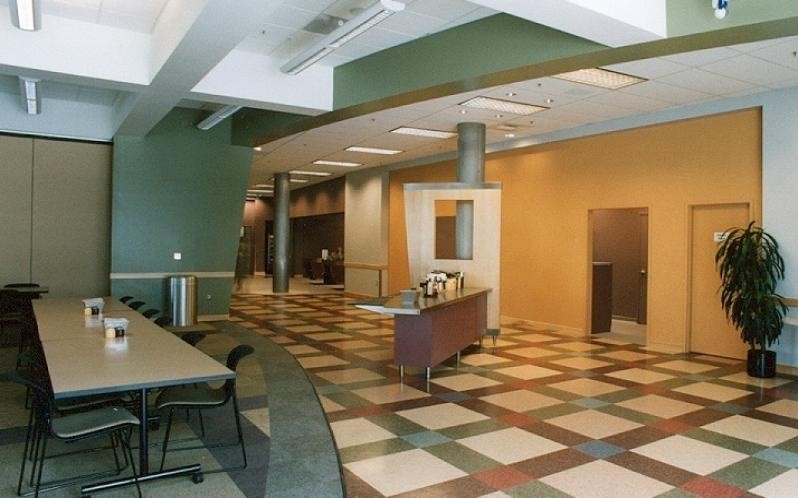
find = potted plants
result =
[713,219,792,379]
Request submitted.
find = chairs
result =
[112,296,208,450]
[153,344,254,480]
[2,296,136,463]
[2,369,142,497]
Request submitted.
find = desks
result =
[30,298,238,498]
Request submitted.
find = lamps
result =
[19,76,42,114]
[8,0,42,32]
[280,0,407,76]
[197,104,245,131]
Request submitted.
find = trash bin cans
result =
[170,275,199,329]
